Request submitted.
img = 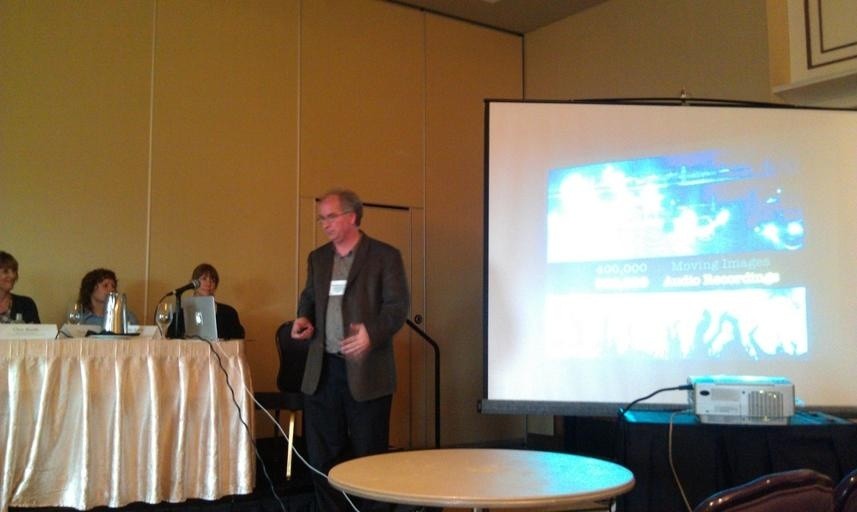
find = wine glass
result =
[67,303,83,339]
[154,303,173,341]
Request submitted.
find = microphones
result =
[165,279,201,296]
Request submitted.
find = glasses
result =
[317,211,353,221]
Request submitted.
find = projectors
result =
[688,374,797,425]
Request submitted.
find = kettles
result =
[103,291,127,335]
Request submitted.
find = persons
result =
[165,263,245,339]
[290,190,409,512]
[66,267,140,326]
[0,250,40,324]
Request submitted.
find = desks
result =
[327,447,639,512]
[615,408,856,512]
[0,334,263,511]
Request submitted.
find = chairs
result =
[250,321,313,480]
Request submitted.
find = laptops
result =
[183,296,244,340]
